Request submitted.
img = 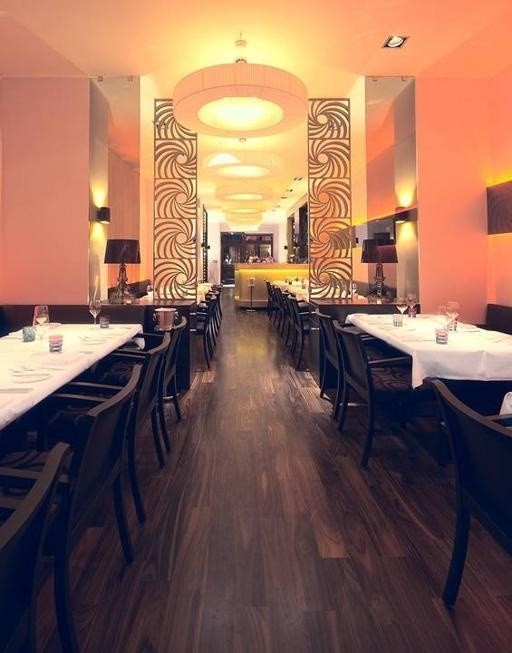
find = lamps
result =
[360,237,401,303]
[392,204,411,225]
[93,204,113,224]
[198,148,282,228]
[102,235,146,303]
[170,29,309,142]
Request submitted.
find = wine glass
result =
[89,300,102,329]
[35,305,50,338]
[446,301,458,333]
[406,292,417,320]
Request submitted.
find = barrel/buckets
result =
[152,307,179,331]
[247,278,256,287]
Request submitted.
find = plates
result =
[12,373,53,383]
[81,337,108,345]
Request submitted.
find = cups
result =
[445,317,457,331]
[408,307,416,318]
[49,333,63,352]
[396,296,407,315]
[22,325,36,341]
[100,314,110,328]
[147,285,154,296]
[434,328,447,343]
[393,313,402,327]
[284,276,312,292]
[349,283,357,293]
